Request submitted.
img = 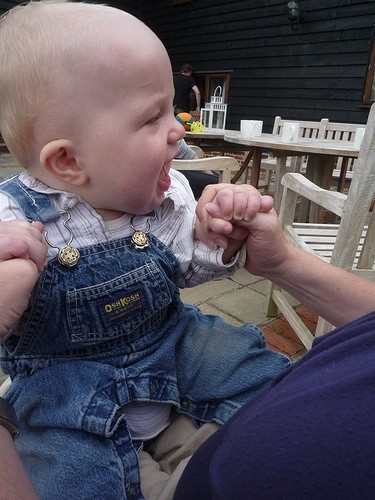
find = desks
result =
[224,132,361,223]
[183,128,235,156]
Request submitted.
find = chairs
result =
[170,101,375,351]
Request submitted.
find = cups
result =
[282,123,299,143]
[255,120,263,136]
[355,128,365,147]
[241,120,256,138]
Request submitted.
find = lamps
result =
[288,0,300,22]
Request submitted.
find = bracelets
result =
[0,397,19,441]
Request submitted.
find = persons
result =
[0,183,375,500]
[0,0,295,500]
